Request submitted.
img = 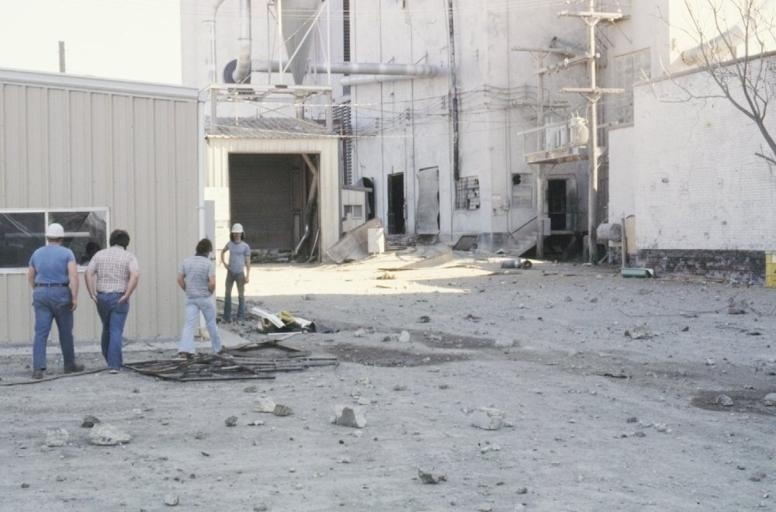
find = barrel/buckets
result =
[764,250,776,288]
[596,223,625,243]
[367,227,385,254]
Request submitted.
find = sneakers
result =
[64,364,83,373]
[32,369,43,379]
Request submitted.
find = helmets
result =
[231,223,244,233]
[45,222,65,238]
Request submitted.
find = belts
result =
[35,283,67,287]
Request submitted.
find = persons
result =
[174,239,224,362]
[27,222,85,379]
[83,229,139,371]
[220,224,251,321]
